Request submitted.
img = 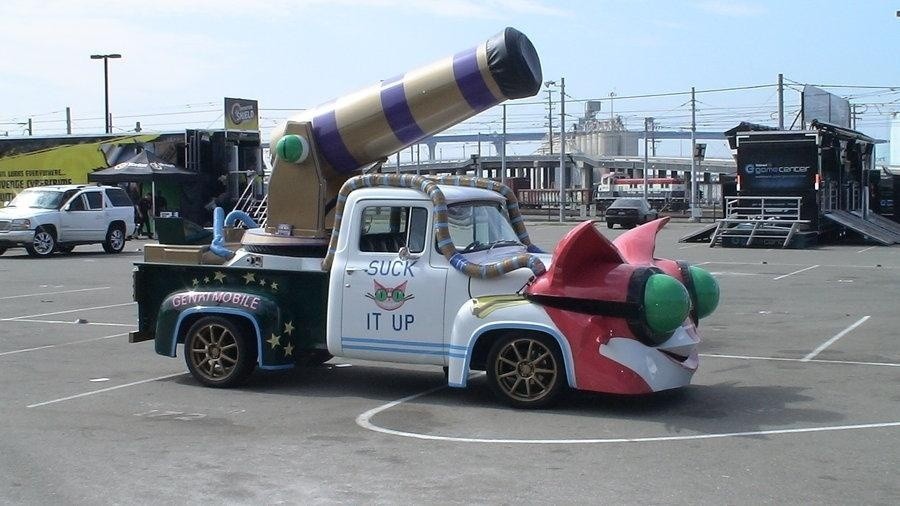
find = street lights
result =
[92,54,123,135]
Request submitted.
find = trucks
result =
[598,172,688,211]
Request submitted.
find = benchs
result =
[361,232,407,252]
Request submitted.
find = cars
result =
[606,197,658,229]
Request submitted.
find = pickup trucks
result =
[132,171,721,413]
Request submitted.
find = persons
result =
[153,189,168,216]
[134,192,155,239]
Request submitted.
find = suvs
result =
[0,184,135,259]
